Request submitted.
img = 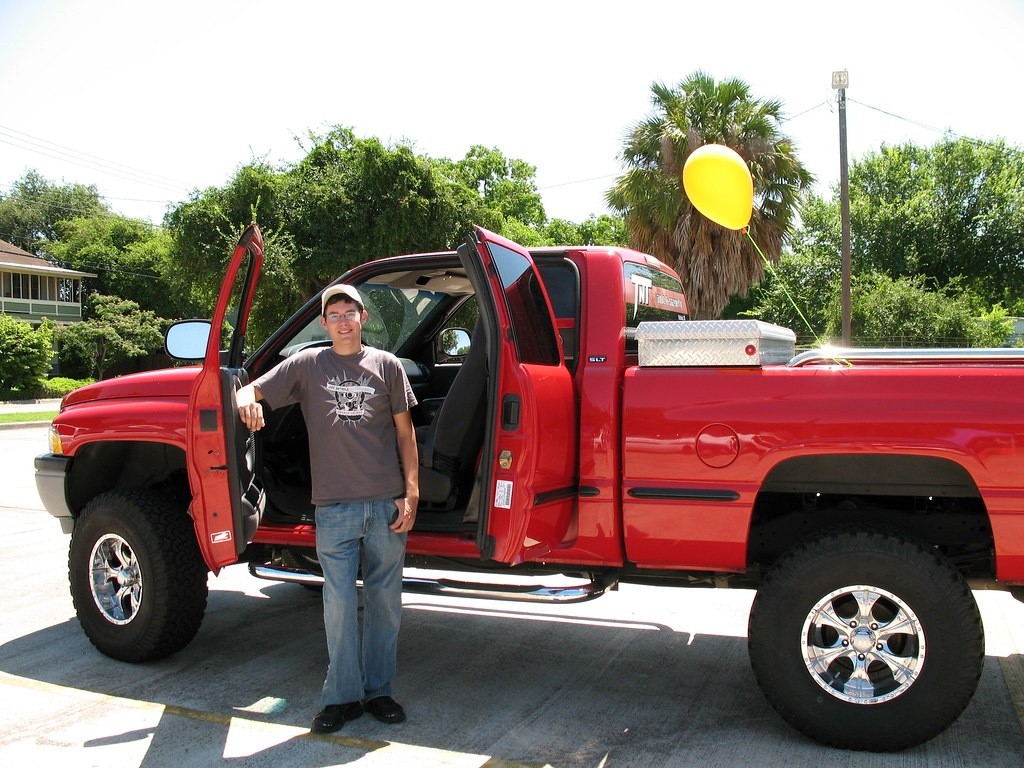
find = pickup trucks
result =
[32,220,1024,752]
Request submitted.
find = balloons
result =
[683,145,753,234]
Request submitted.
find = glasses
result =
[324,312,362,323]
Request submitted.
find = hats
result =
[321,283,364,315]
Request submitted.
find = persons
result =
[237,284,420,732]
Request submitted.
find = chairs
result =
[397,310,488,514]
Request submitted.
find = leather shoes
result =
[311,701,364,734]
[365,696,406,724]
